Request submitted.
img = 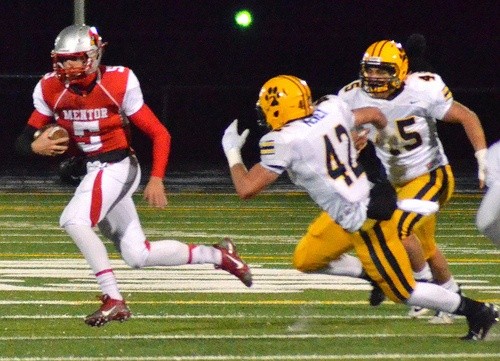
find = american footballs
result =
[32,125,68,148]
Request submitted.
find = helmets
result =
[50,23,108,81]
[256,75,315,130]
[358,39,409,99]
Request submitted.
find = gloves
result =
[220,118,250,169]
[372,125,392,148]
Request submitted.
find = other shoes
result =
[407,277,435,318]
[428,284,466,324]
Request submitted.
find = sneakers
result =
[84,293,132,328]
[213,237,253,287]
[459,302,499,341]
[369,280,386,306]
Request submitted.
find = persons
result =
[221,74,498,341]
[13,23,252,326]
[337,39,487,324]
